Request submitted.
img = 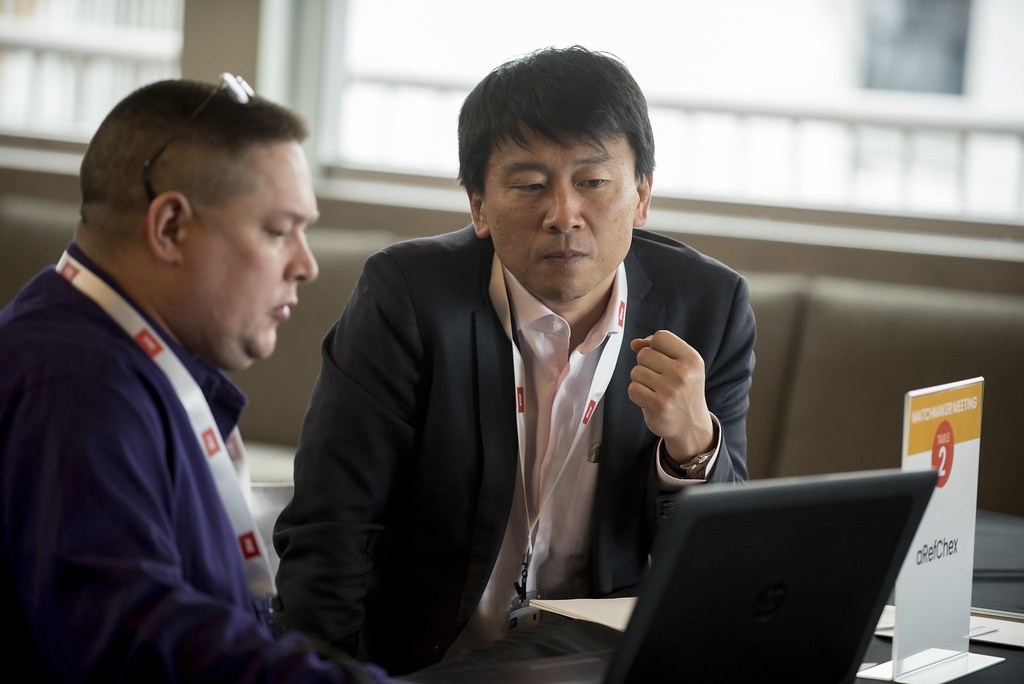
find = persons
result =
[0,72,392,684]
[267,45,753,684]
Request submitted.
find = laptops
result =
[407,466,940,684]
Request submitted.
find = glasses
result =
[141,72,254,203]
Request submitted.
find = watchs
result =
[660,436,715,480]
